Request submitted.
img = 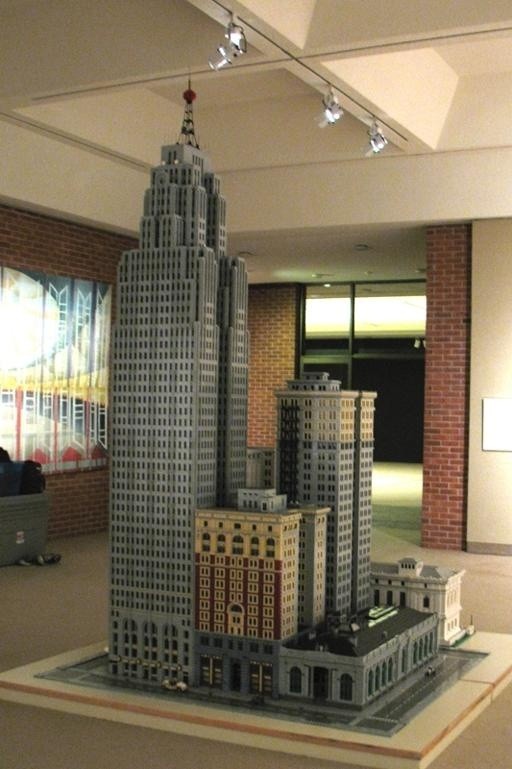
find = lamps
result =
[209,6,249,72]
[314,82,344,131]
[363,115,389,162]
